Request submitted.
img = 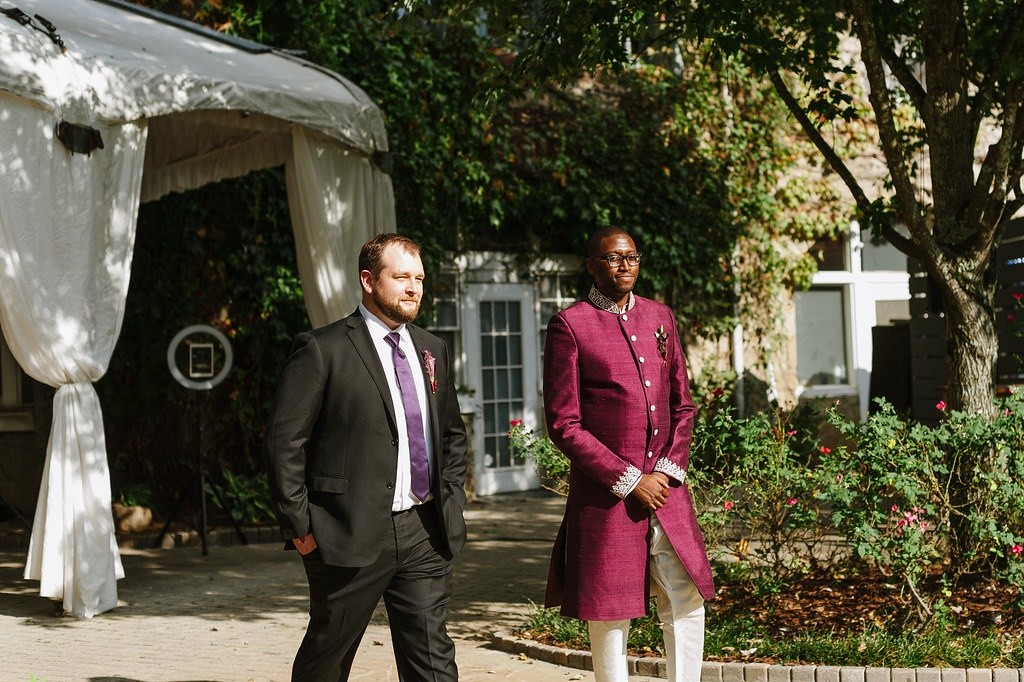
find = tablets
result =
[189,344,214,379]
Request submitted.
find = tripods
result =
[149,381,248,557]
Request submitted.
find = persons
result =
[265,233,469,682]
[542,226,716,681]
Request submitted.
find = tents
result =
[1,0,396,620]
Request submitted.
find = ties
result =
[383,332,431,503]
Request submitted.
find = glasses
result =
[593,253,642,268]
